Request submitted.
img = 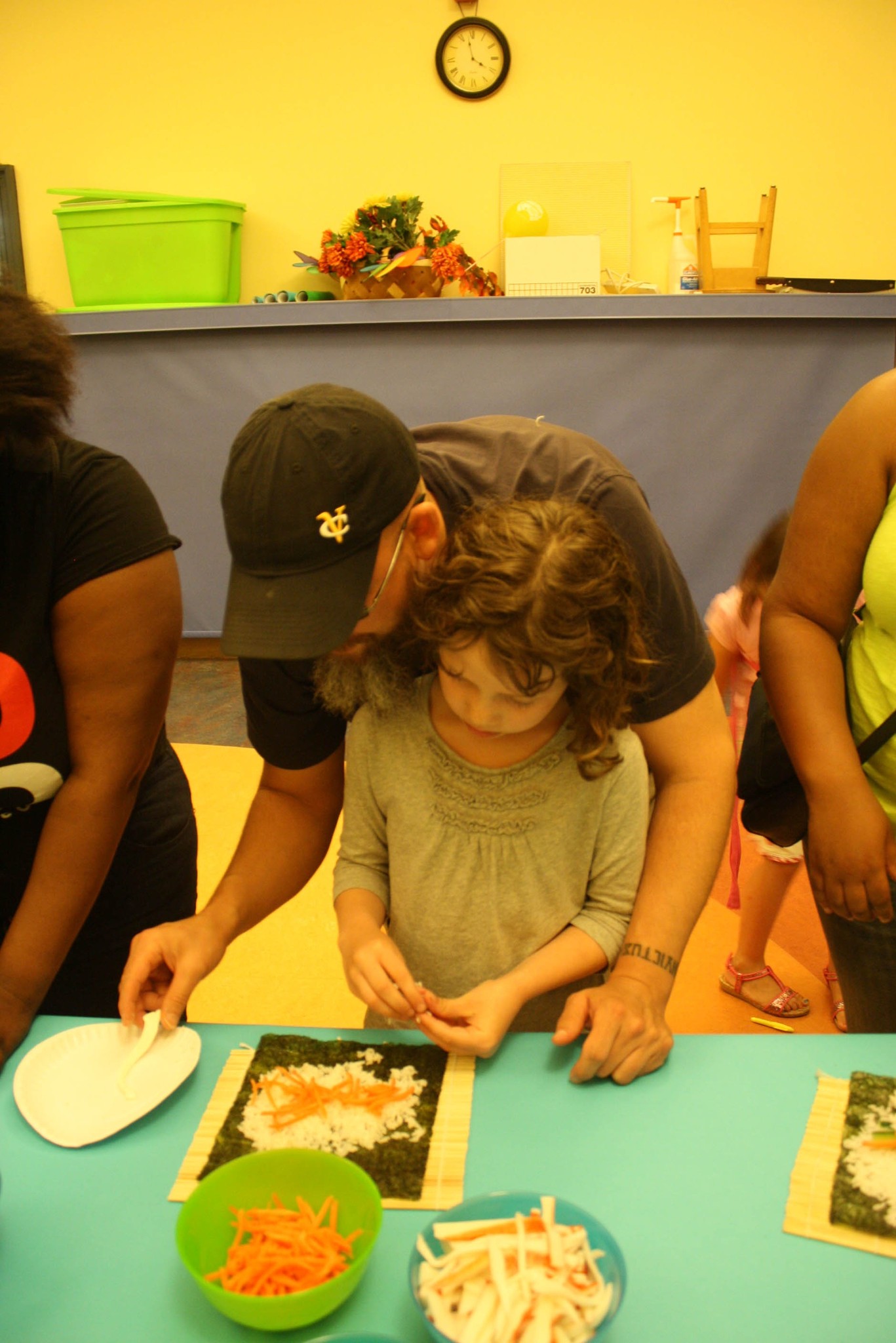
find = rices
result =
[842,1088,896,1229]
[236,1049,428,1160]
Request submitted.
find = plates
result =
[12,1018,201,1147]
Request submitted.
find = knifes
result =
[754,276,895,293]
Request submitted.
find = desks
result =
[0,1014,896,1343]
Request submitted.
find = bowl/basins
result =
[173,1149,382,1333]
[406,1189,626,1342]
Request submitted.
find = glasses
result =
[359,492,426,619]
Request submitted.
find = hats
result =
[222,385,419,658]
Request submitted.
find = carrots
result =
[249,1063,416,1131]
[204,1192,366,1297]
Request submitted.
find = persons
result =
[0,263,198,1071]
[119,383,740,1085]
[759,370,895,1032]
[331,496,650,1059]
[702,505,848,1032]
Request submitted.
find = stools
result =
[695,186,776,293]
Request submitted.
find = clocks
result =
[435,17,511,98]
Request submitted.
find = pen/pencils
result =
[750,1017,794,1033]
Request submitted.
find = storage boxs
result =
[48,189,246,310]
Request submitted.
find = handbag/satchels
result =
[735,647,848,846]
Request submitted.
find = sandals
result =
[820,966,853,1031]
[719,953,810,1016]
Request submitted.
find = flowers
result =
[315,195,502,295]
